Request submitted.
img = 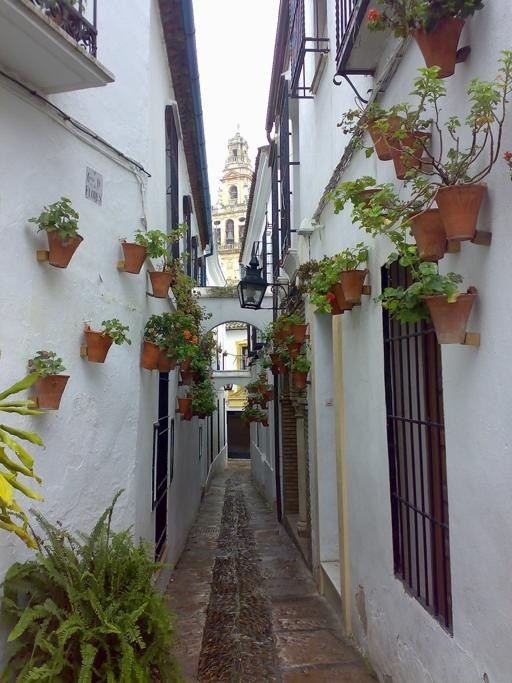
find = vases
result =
[410,15,465,77]
[143,339,178,373]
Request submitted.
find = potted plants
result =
[336,103,409,161]
[264,308,308,389]
[315,254,355,312]
[85,316,131,363]
[26,349,71,409]
[386,65,448,180]
[397,49,512,240]
[315,267,345,316]
[123,218,190,299]
[402,178,447,262]
[239,360,271,426]
[180,329,219,420]
[396,262,479,345]
[26,195,83,268]
[333,176,399,225]
[335,241,369,304]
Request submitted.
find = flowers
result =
[147,309,200,359]
[308,292,336,312]
[365,0,485,47]
